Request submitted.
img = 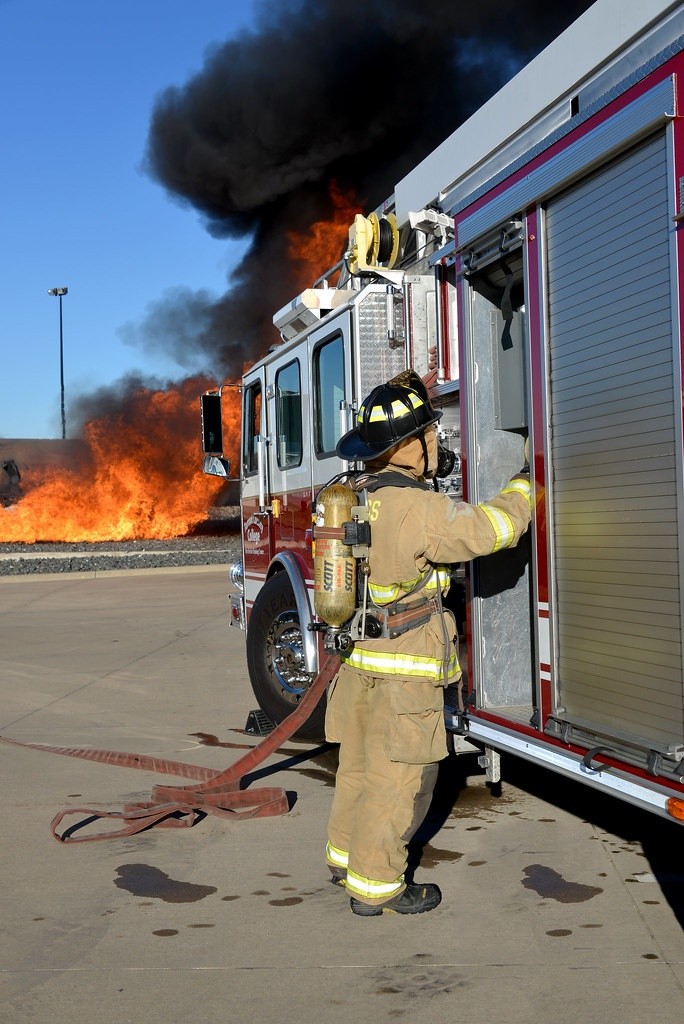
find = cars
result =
[0,459,21,499]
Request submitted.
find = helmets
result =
[336,384,443,462]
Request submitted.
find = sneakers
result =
[327,866,348,888]
[350,878,442,917]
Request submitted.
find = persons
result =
[325,370,531,916]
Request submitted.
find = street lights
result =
[47,286,68,439]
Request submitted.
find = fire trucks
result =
[201,0,682,824]
